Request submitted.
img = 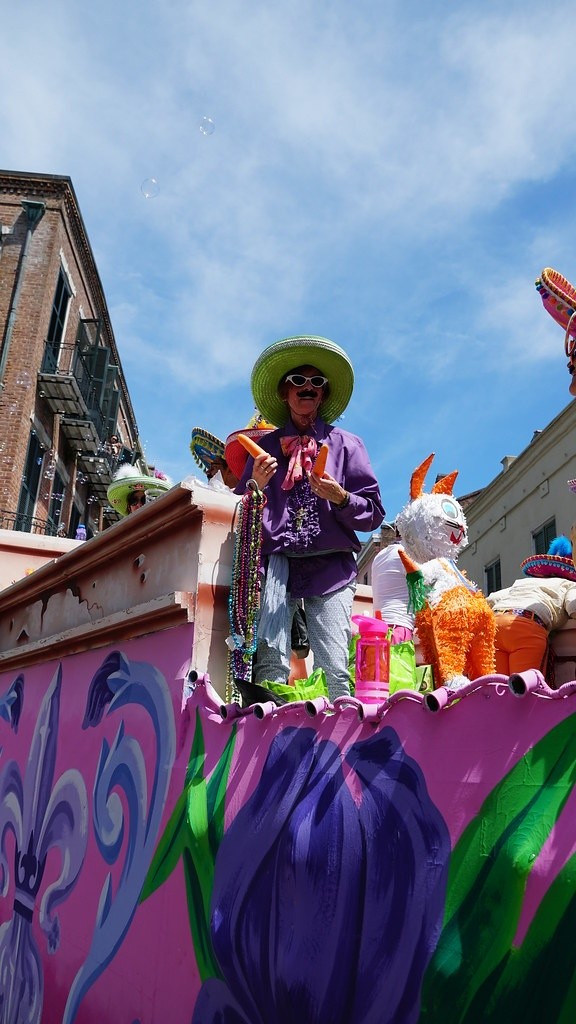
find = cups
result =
[144,489,166,505]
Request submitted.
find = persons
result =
[107,466,172,515]
[110,435,122,454]
[190,426,238,492]
[486,534,576,679]
[372,522,414,643]
[238,335,386,711]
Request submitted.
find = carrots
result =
[237,434,271,464]
[312,444,329,479]
[397,549,417,573]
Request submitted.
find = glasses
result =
[285,375,328,388]
[127,495,145,506]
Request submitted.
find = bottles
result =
[75,523,86,541]
[351,614,391,705]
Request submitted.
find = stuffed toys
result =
[398,452,497,690]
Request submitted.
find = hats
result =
[107,463,172,516]
[190,427,226,477]
[225,407,279,480]
[250,335,354,428]
[519,536,576,582]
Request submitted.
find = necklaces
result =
[294,446,320,526]
[225,490,263,705]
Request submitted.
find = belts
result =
[493,609,547,631]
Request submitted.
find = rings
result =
[315,488,318,491]
[319,483,321,485]
[260,465,265,470]
[265,470,268,473]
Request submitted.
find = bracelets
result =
[338,493,349,509]
